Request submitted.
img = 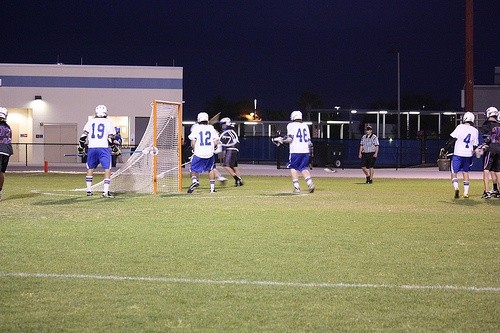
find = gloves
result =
[113,144,121,155]
[78,147,86,156]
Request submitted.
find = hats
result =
[365,125,372,129]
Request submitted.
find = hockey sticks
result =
[64,146,159,157]
[157,161,191,180]
[274,138,338,173]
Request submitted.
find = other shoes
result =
[366,175,373,183]
[493,192,500,198]
[481,190,491,198]
[217,176,227,182]
[236,177,243,186]
[309,184,315,193]
[489,188,498,194]
[292,187,300,193]
[463,192,469,197]
[454,189,460,199]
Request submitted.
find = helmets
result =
[462,111,475,124]
[484,106,499,119]
[290,109,303,121]
[115,126,120,134]
[0,106,9,118]
[219,117,232,130]
[95,104,108,117]
[197,111,209,123]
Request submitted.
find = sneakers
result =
[87,190,93,195]
[102,191,114,198]
[188,181,200,193]
[209,188,216,192]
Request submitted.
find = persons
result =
[108,133,122,167]
[78,104,115,198]
[209,112,227,184]
[475,107,500,198]
[79,134,88,164]
[0,107,13,200]
[274,111,315,194]
[359,125,380,184]
[489,111,500,195]
[188,112,219,193]
[450,112,479,199]
[218,117,243,186]
